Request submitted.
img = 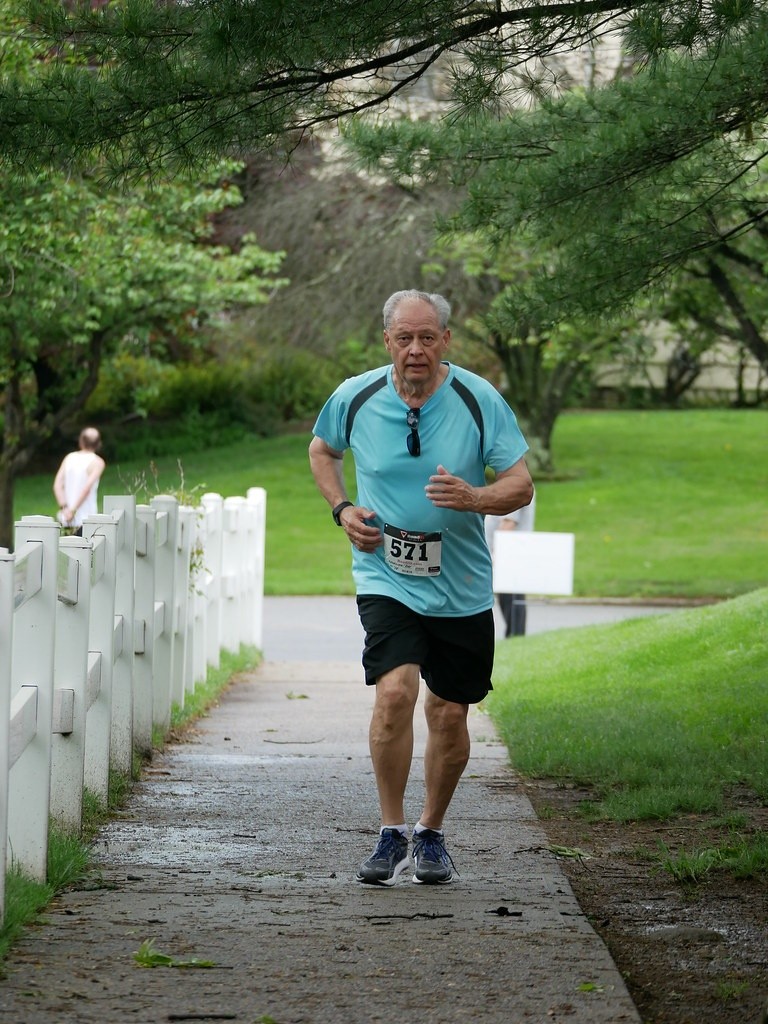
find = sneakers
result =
[410,829,453,885]
[356,829,411,887]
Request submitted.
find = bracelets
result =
[60,504,67,510]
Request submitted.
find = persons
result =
[483,458,537,639]
[51,425,105,537]
[305,287,535,889]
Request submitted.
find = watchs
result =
[331,501,353,526]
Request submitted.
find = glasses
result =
[406,407,421,458]
